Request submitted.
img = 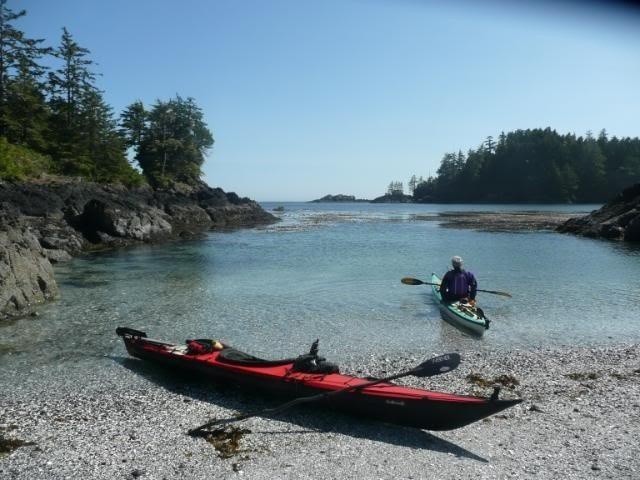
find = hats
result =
[453,256,462,264]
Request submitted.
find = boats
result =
[432,272,492,331]
[116,327,524,431]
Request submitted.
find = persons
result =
[440,256,477,306]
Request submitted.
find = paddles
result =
[402,275,512,301]
[188,354,460,436]
